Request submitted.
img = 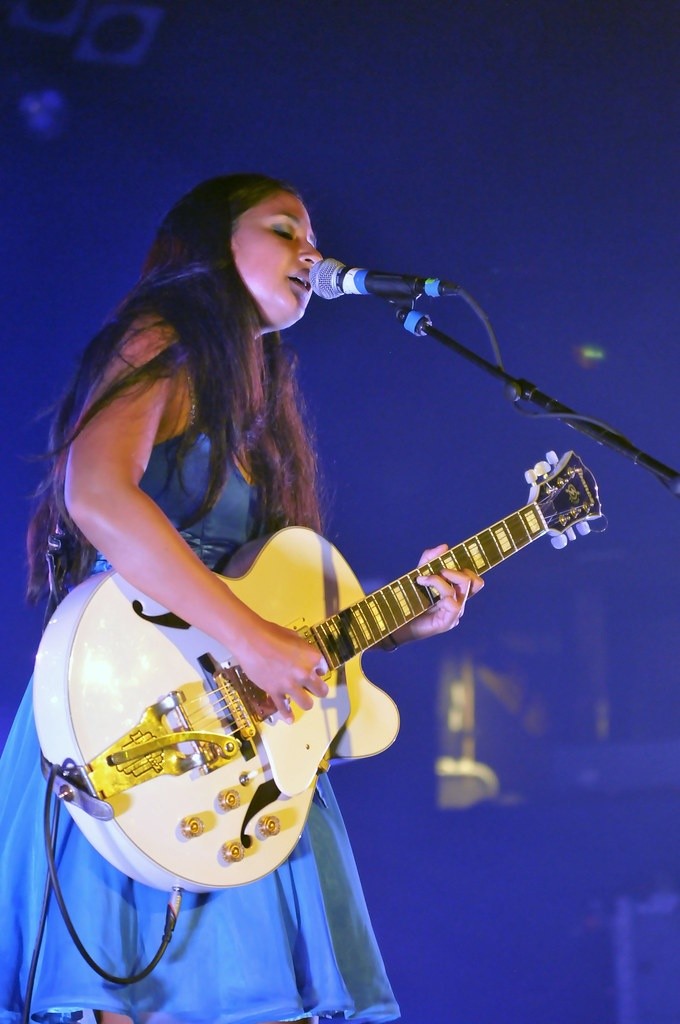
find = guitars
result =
[33,447,600,893]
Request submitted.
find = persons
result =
[0,171,485,1024]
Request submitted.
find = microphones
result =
[309,259,459,299]
